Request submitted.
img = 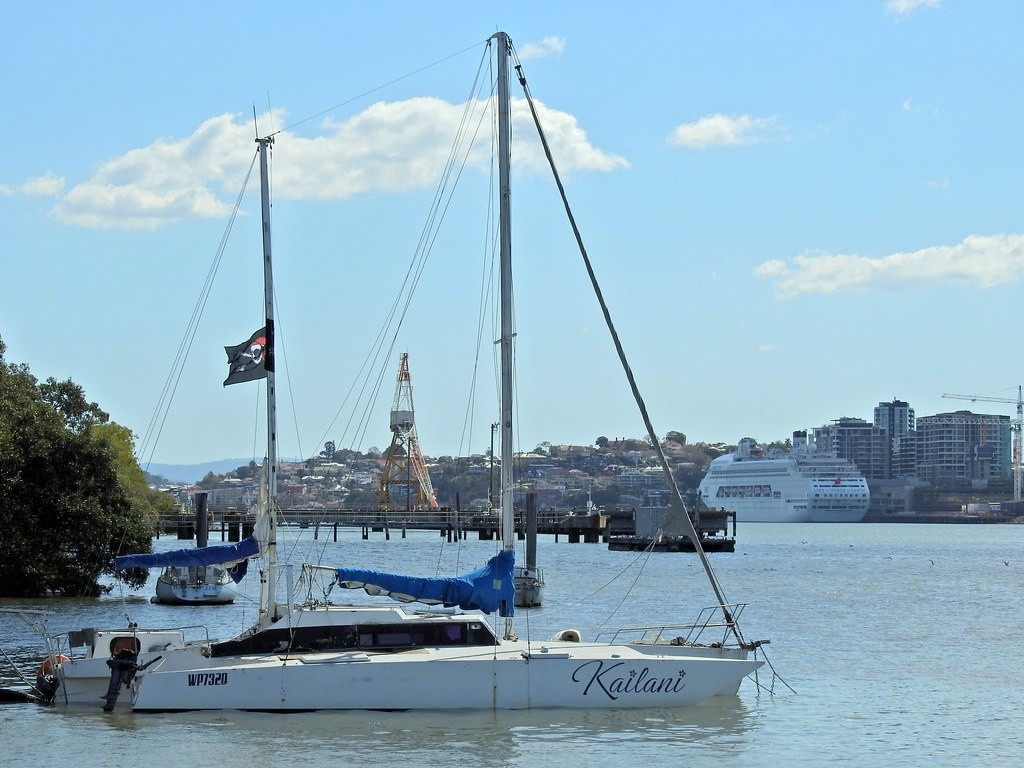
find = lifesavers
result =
[39,656,71,675]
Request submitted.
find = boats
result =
[695,437,871,523]
[508,563,546,608]
[154,562,238,606]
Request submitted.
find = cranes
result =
[941,386,1024,501]
[377,351,441,511]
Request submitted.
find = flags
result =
[223,318,275,388]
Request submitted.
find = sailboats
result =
[0,30,775,718]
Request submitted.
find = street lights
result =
[488,421,501,516]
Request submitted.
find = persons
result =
[616,506,618,511]
[621,508,623,511]
